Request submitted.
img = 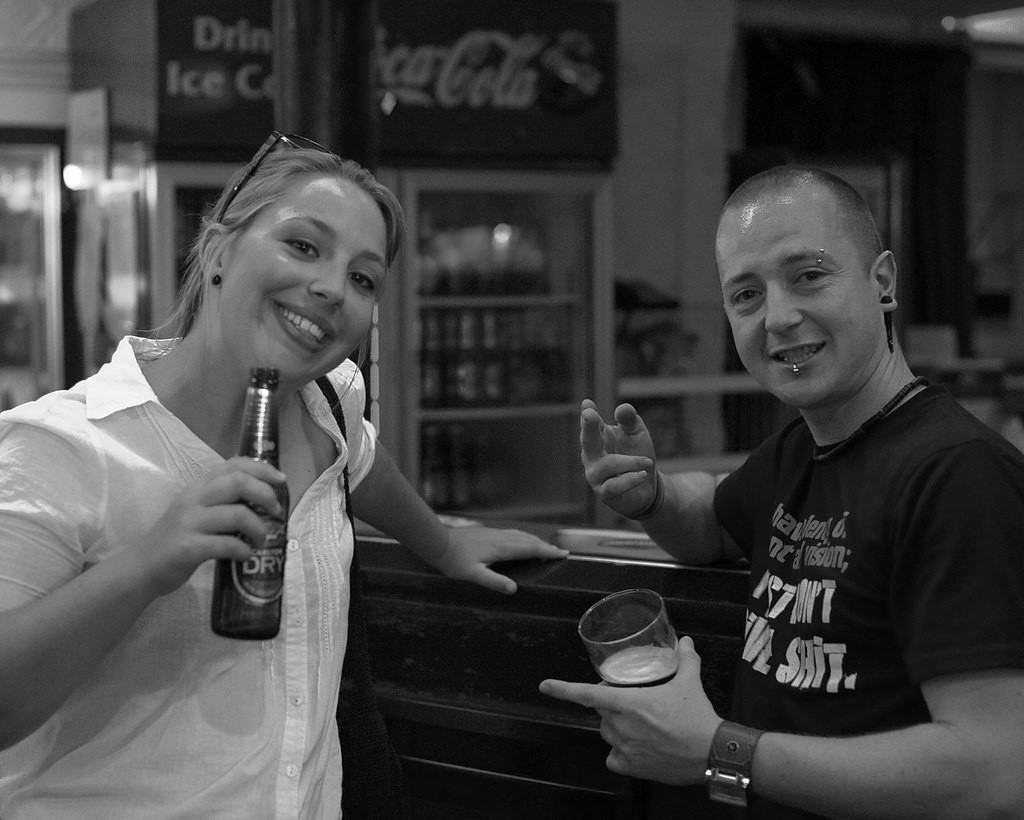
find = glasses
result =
[215,130,347,226]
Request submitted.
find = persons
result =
[539,166,1021,820]
[1,147,567,820]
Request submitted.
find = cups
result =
[579,589,678,687]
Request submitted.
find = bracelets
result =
[704,720,766,810]
[631,469,666,522]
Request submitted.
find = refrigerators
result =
[67,0,616,547]
[0,124,83,414]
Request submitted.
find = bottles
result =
[424,425,474,511]
[211,367,290,639]
[421,312,563,408]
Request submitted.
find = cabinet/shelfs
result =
[399,171,609,537]
